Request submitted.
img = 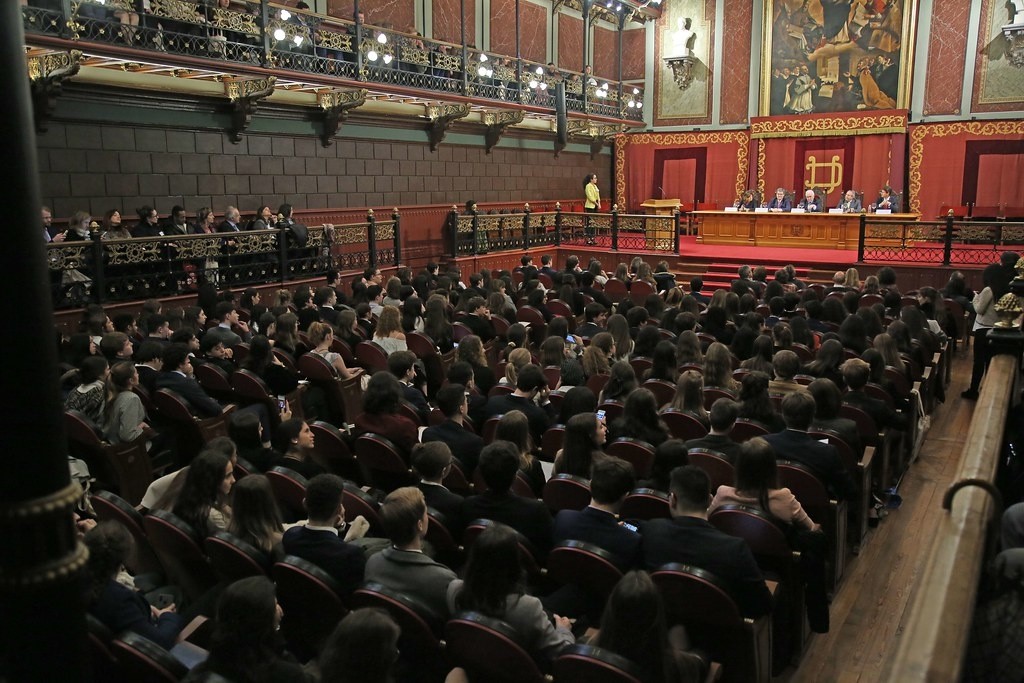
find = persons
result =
[799,189,822,212]
[66,206,109,304]
[463,200,489,253]
[253,205,279,283]
[1000,252,1021,281]
[961,262,1011,400]
[39,205,71,309]
[995,501,1024,592]
[135,206,177,296]
[22,1,595,114]
[45,248,972,683]
[277,203,297,231]
[194,208,235,291]
[98,207,135,303]
[165,206,200,292]
[768,187,792,212]
[836,190,862,213]
[583,173,602,243]
[738,191,757,210]
[216,204,252,287]
[877,185,899,213]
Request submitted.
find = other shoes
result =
[962,389,979,400]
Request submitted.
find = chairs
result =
[804,186,827,213]
[48,206,980,683]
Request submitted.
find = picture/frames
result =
[759,0,917,116]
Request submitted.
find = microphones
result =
[658,186,667,199]
[809,202,811,213]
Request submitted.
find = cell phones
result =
[157,594,174,609]
[101,232,107,240]
[566,334,576,343]
[623,522,639,532]
[63,230,68,235]
[277,394,286,413]
[597,409,606,420]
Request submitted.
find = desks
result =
[692,209,920,250]
